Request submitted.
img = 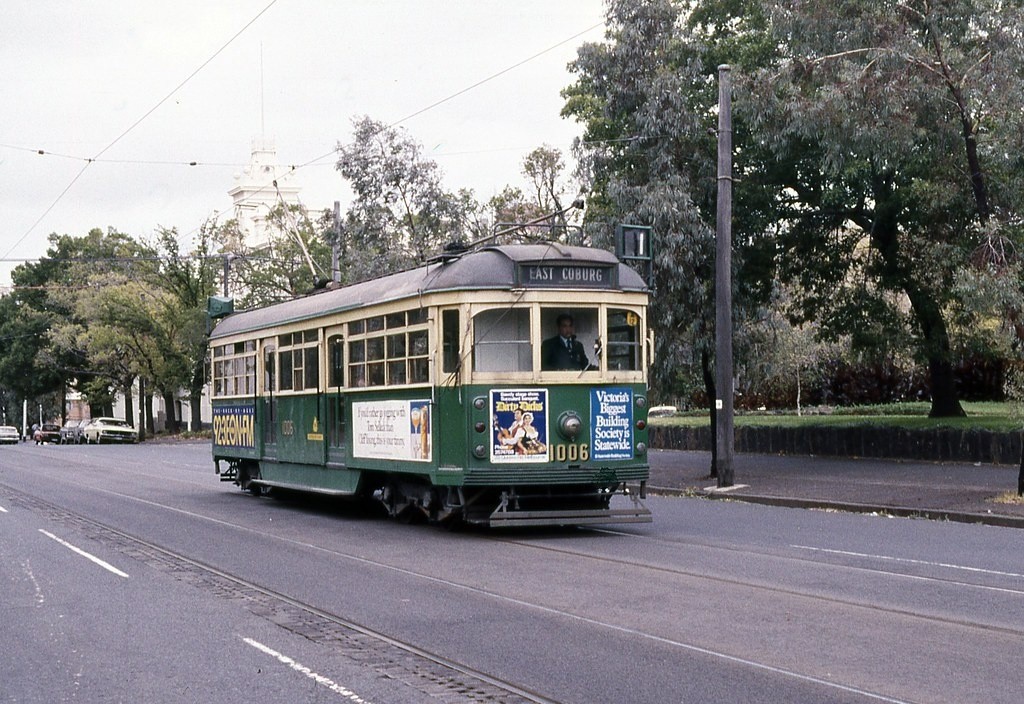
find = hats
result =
[522,412,534,423]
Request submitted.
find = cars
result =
[84,417,137,444]
[0,426,20,444]
[60,419,91,444]
[34,424,62,445]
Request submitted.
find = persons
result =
[356,353,385,386]
[541,314,600,371]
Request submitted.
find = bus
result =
[208,201,655,527]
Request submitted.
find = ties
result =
[567,339,572,358]
